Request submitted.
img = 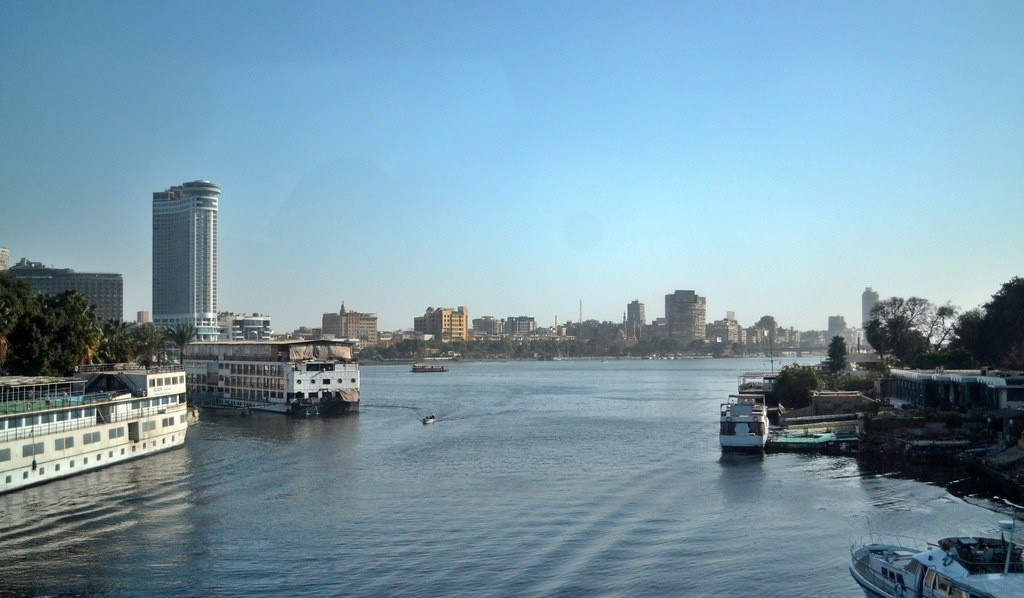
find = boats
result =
[764,423,1024,510]
[183,338,360,419]
[409,364,449,373]
[848,511,1024,598]
[0,362,188,495]
[737,370,780,405]
[422,414,437,425]
[718,394,768,451]
